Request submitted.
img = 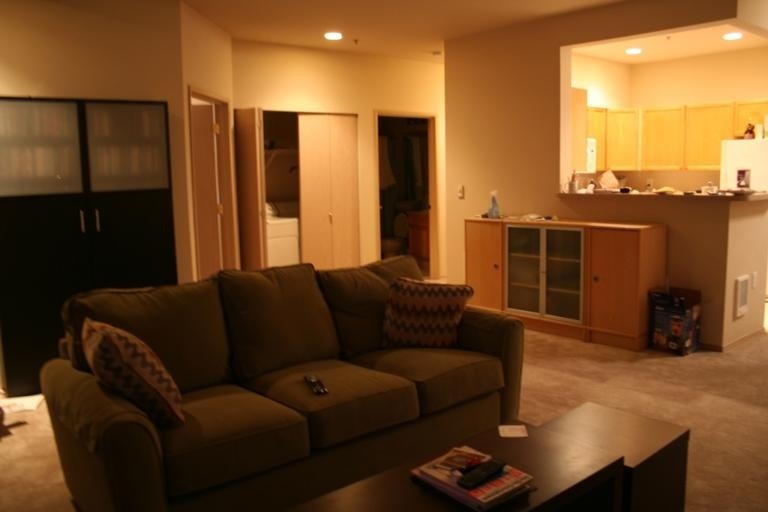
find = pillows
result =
[80,315,189,433]
[375,273,476,347]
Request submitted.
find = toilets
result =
[382,212,409,259]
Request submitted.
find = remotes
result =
[304,372,328,395]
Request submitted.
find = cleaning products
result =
[487,189,500,218]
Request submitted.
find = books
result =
[411,445,536,512]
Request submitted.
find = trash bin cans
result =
[649,286,702,357]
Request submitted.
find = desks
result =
[538,400,689,511]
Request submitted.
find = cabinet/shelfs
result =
[568,88,768,178]
[463,214,664,354]
[0,92,178,398]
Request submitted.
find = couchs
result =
[36,250,525,510]
